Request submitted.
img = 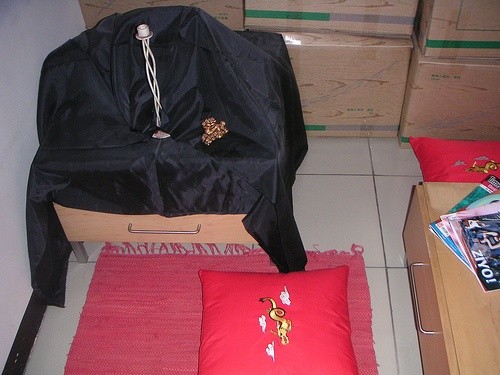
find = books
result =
[429,175,500,293]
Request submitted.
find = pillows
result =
[407,136,500,182]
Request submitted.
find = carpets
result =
[196,265,359,375]
[63,241,380,375]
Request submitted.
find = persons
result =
[474,232,500,249]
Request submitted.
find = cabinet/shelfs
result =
[52,201,259,263]
[402,181,500,375]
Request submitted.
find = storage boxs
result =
[80,0,500,149]
